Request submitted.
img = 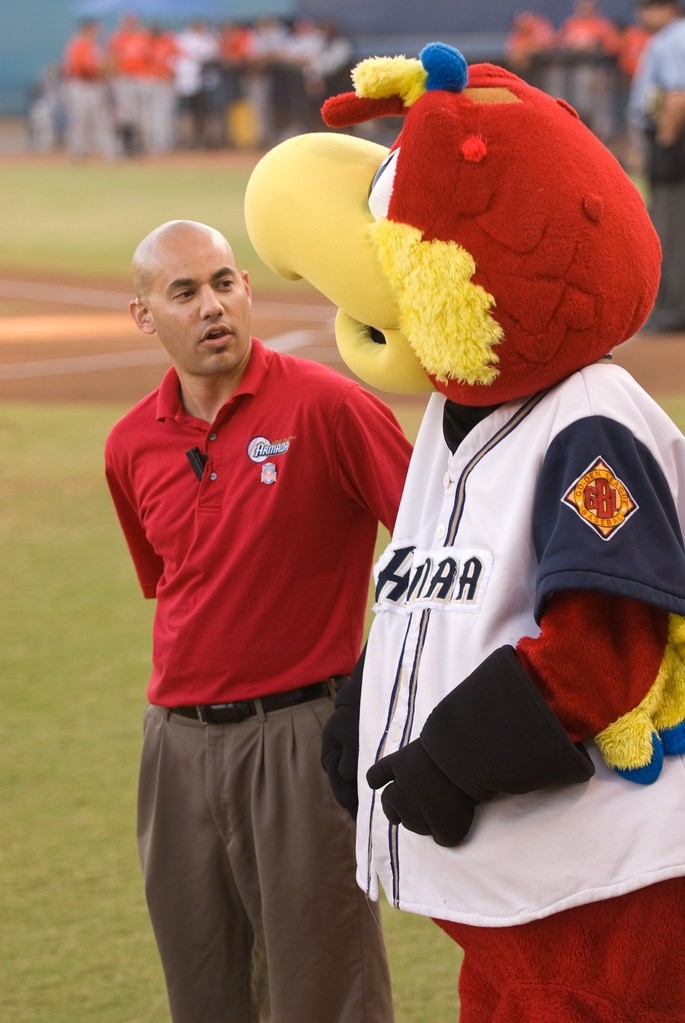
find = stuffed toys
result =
[242,34,685,1023]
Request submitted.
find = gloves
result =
[650,144,681,187]
[319,645,366,819]
[366,645,596,848]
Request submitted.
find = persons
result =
[502,1,685,155]
[43,10,359,160]
[623,1,685,338]
[105,216,415,1023]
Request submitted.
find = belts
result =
[167,674,351,727]
